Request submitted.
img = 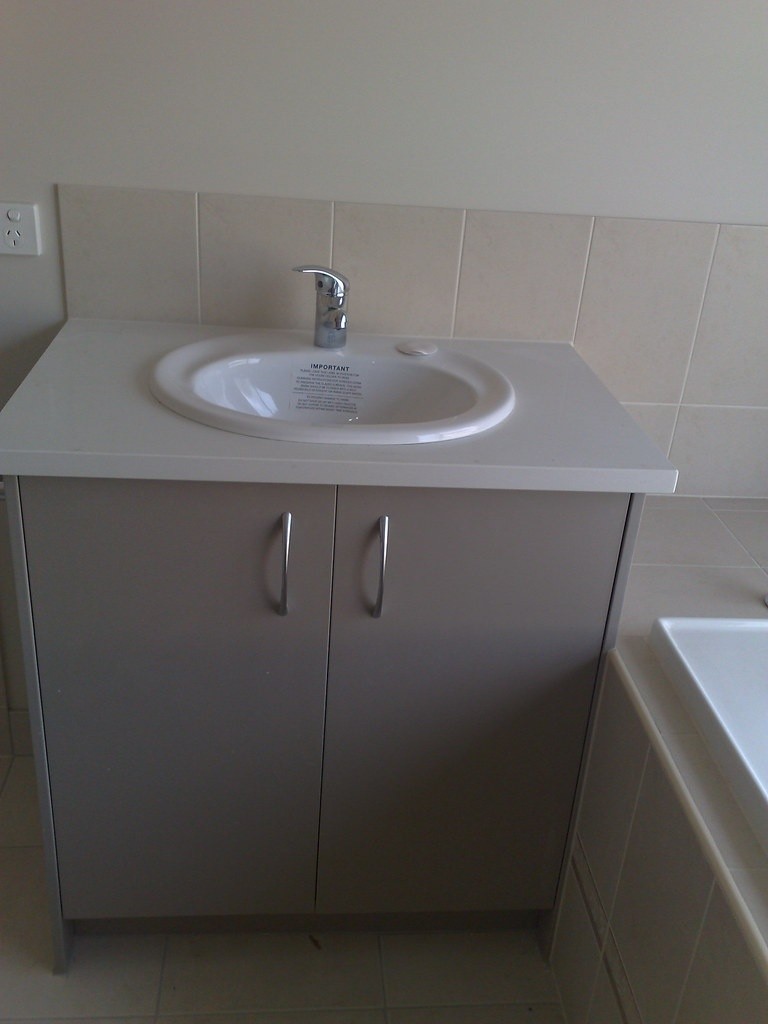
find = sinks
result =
[148,334,516,444]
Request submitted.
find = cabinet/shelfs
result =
[3,476,648,974]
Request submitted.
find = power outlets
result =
[0,202,43,257]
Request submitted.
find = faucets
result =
[292,265,347,348]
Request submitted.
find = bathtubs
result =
[547,496,768,1024]
